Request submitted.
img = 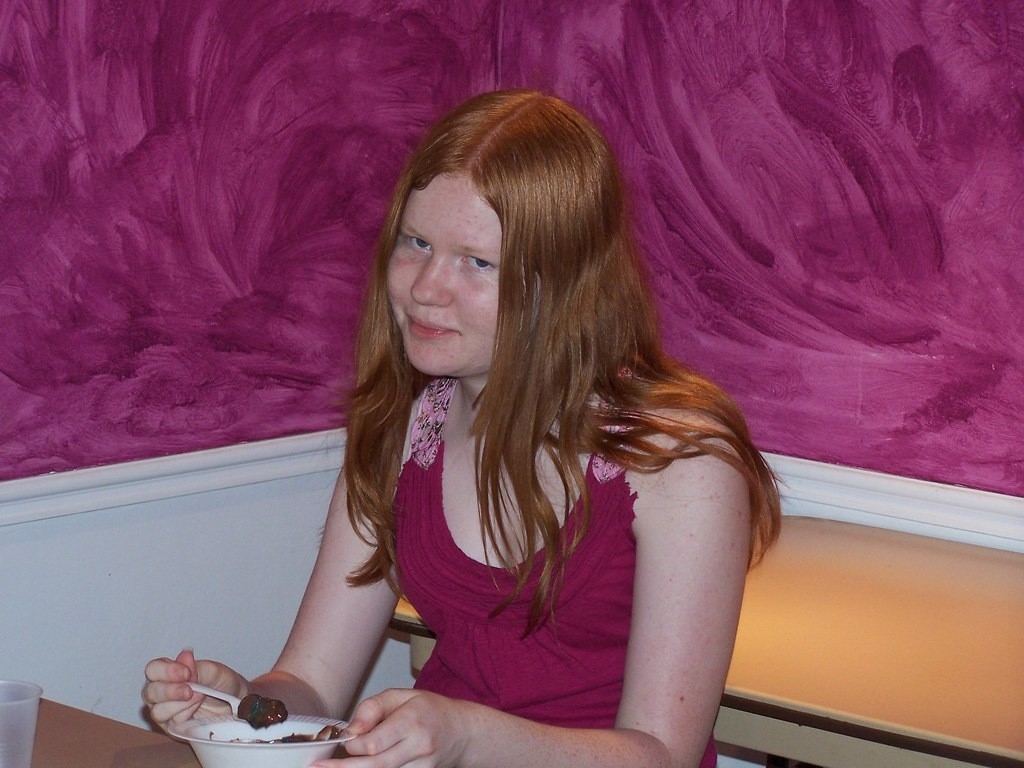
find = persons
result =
[143,89,780,768]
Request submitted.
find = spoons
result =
[185,682,250,723]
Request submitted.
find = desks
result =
[388,514,1024,768]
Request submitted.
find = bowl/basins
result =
[167,714,358,768]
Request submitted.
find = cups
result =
[0,680,44,768]
